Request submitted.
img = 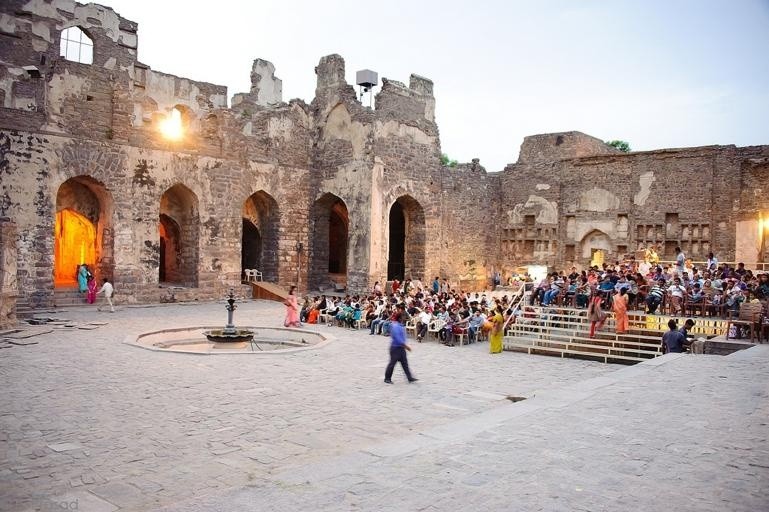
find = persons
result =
[78,264,91,291]
[95,278,115,312]
[87,274,97,303]
[382,312,420,383]
[284,274,525,356]
[525,245,768,355]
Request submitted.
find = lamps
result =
[244,268,262,282]
[356,315,367,330]
[726,302,762,343]
[682,290,727,318]
[404,321,417,339]
[318,309,329,324]
[451,325,484,347]
[427,320,447,342]
